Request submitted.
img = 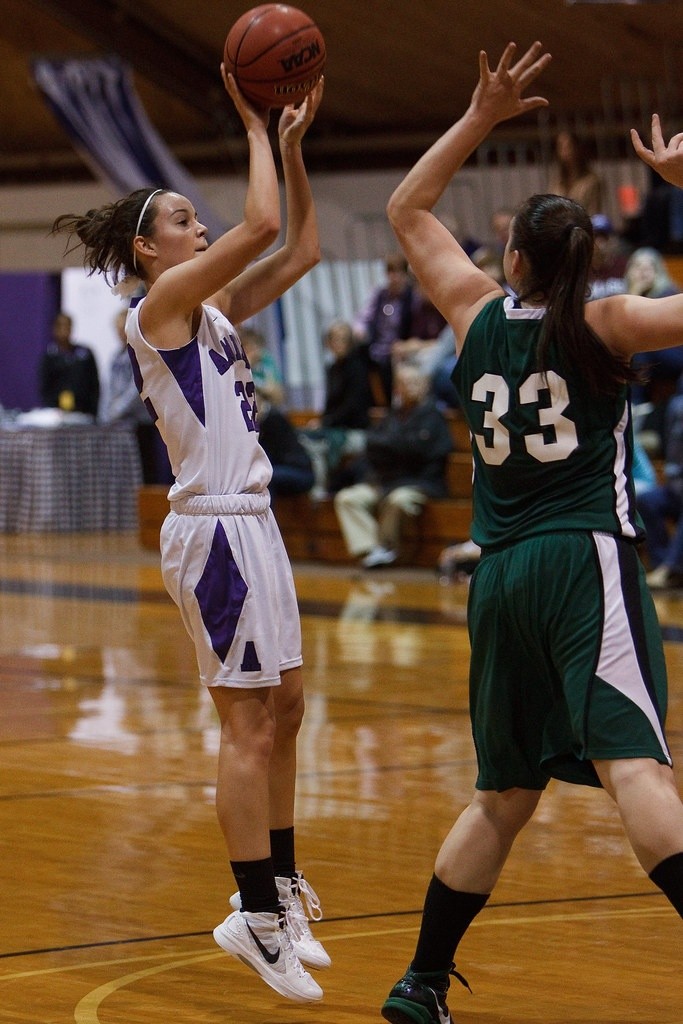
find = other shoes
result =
[362,547,398,568]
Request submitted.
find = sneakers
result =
[381,965,456,1023]
[212,870,332,1002]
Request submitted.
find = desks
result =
[0,410,140,538]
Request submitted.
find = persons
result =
[237,206,519,584]
[52,62,332,1004]
[382,40,683,1024]
[549,125,683,587]
[39,315,100,420]
[107,310,175,486]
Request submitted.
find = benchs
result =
[134,401,476,575]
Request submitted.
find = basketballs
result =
[223,2,328,105]
